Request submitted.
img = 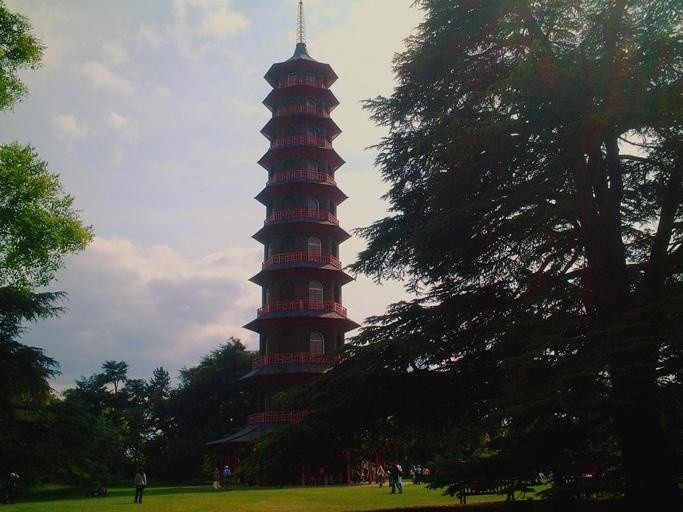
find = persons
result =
[222,465,231,478]
[211,466,221,490]
[12,473,19,503]
[133,465,146,504]
[96,479,107,497]
[4,470,15,503]
[352,461,430,494]
